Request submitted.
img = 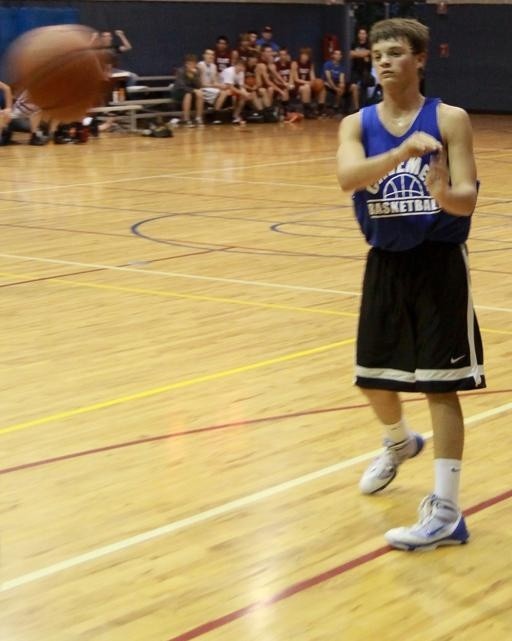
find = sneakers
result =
[385,496,468,552]
[359,432,424,495]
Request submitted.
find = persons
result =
[1,30,132,146]
[333,16,487,554]
[174,27,425,128]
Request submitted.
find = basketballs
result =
[311,78,324,91]
[246,77,255,86]
[7,24,116,124]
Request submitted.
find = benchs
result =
[111,77,208,136]
